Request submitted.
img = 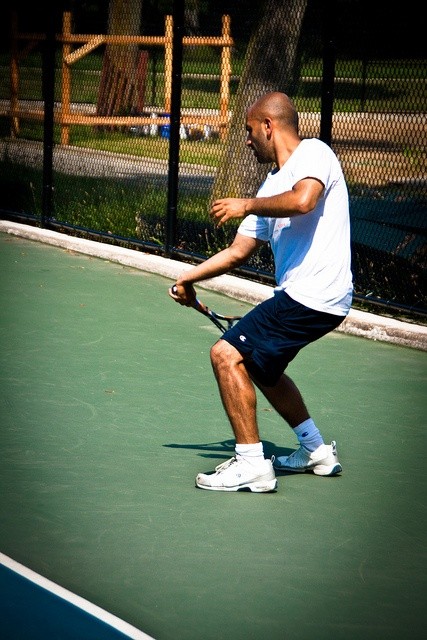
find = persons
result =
[176,90,356,493]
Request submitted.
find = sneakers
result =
[273,441,343,476]
[196,455,278,493]
